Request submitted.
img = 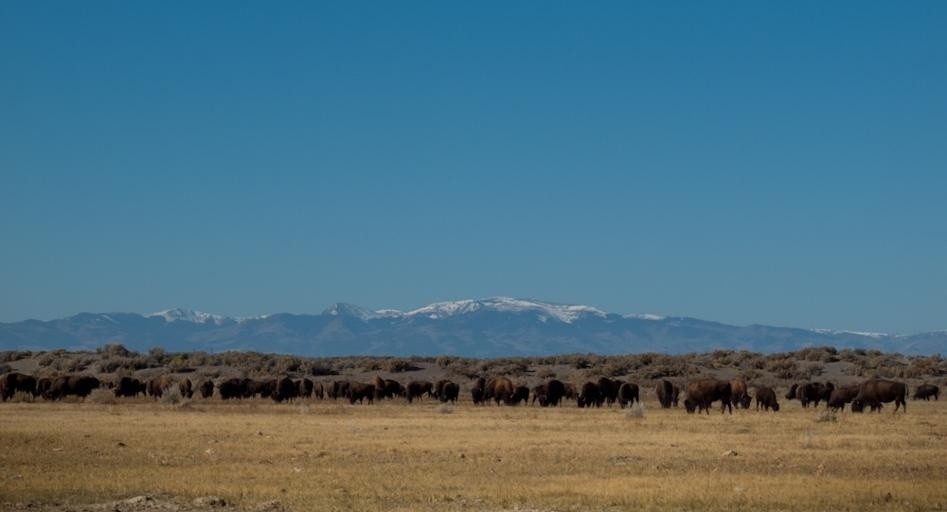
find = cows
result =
[755,387,780,411]
[179,378,195,398]
[785,373,910,415]
[200,374,459,405]
[471,377,639,409]
[914,384,940,401]
[655,376,752,416]
[0,373,172,402]
